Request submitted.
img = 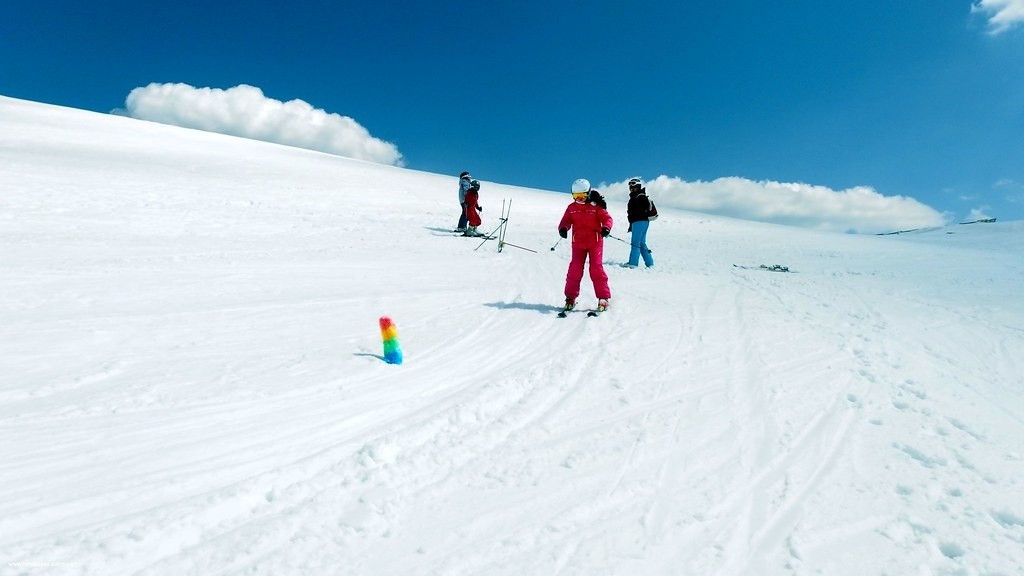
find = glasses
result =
[573,193,588,202]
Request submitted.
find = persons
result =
[464,180,484,236]
[558,179,613,311]
[623,179,654,269]
[457,172,472,231]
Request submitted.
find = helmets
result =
[571,178,591,194]
[628,179,642,190]
[460,172,471,179]
[471,179,481,188]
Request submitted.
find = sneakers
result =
[457,227,467,232]
[565,303,575,310]
[598,298,609,312]
[467,227,485,236]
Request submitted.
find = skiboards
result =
[734,260,799,274]
[559,299,608,321]
[428,228,497,244]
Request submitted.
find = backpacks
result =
[648,199,659,221]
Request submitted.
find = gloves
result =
[601,227,610,237]
[559,227,568,239]
[464,205,468,208]
[477,206,482,211]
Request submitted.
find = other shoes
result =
[645,265,655,270]
[622,263,637,269]
